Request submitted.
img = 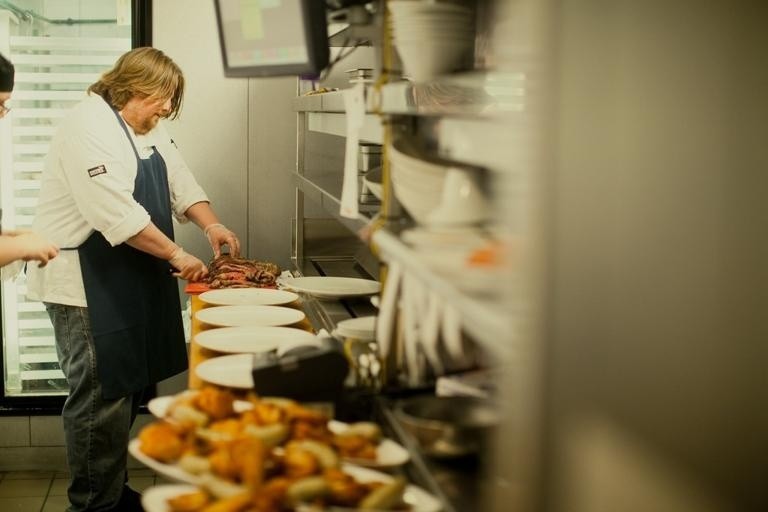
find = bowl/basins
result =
[385,0,474,77]
[391,397,502,461]
[368,140,488,223]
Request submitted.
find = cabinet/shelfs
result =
[289,74,533,512]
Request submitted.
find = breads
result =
[137,387,416,511]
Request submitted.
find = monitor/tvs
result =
[213,0,330,77]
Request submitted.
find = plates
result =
[128,436,440,511]
[141,486,206,511]
[196,355,256,388]
[334,316,377,342]
[194,327,317,354]
[281,278,382,299]
[147,394,409,468]
[198,288,299,306]
[194,307,304,328]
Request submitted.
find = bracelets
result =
[169,246,183,264]
[203,224,225,234]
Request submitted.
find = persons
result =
[24,48,241,512]
[0,54,59,268]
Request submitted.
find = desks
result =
[188,290,316,391]
